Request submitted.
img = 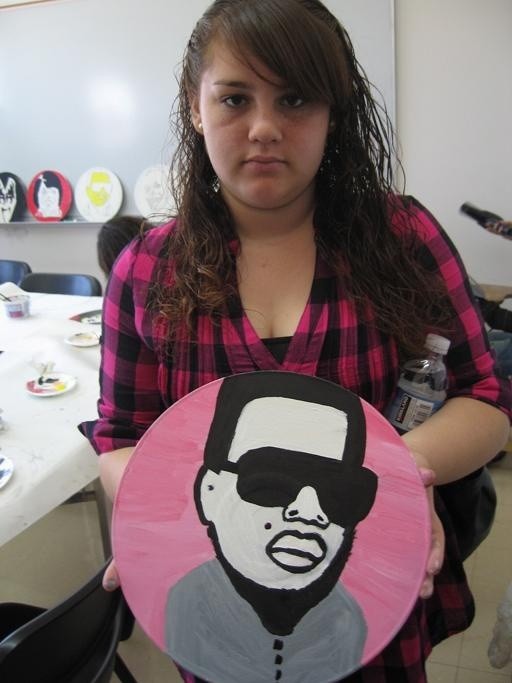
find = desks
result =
[0,282,107,560]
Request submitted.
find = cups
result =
[2,295,30,321]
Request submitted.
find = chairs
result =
[1,258,32,288]
[0,562,136,683]
[17,271,106,293]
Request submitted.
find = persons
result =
[77,0,512,682]
[485,220,512,241]
[98,216,157,275]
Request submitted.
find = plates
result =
[25,372,78,397]
[0,452,16,490]
[63,330,101,348]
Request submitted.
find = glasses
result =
[215,445,381,532]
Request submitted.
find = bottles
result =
[383,333,451,436]
